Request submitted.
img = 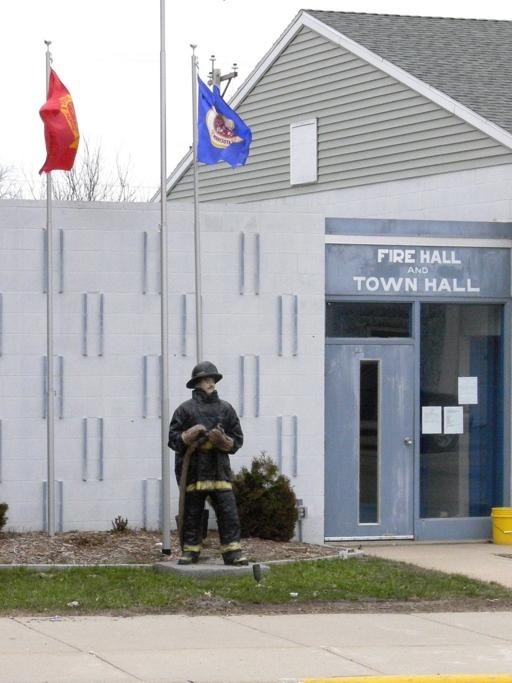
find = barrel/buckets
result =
[491,507,512,545]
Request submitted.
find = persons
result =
[167,360,251,567]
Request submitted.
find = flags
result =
[195,73,252,169]
[37,68,80,174]
